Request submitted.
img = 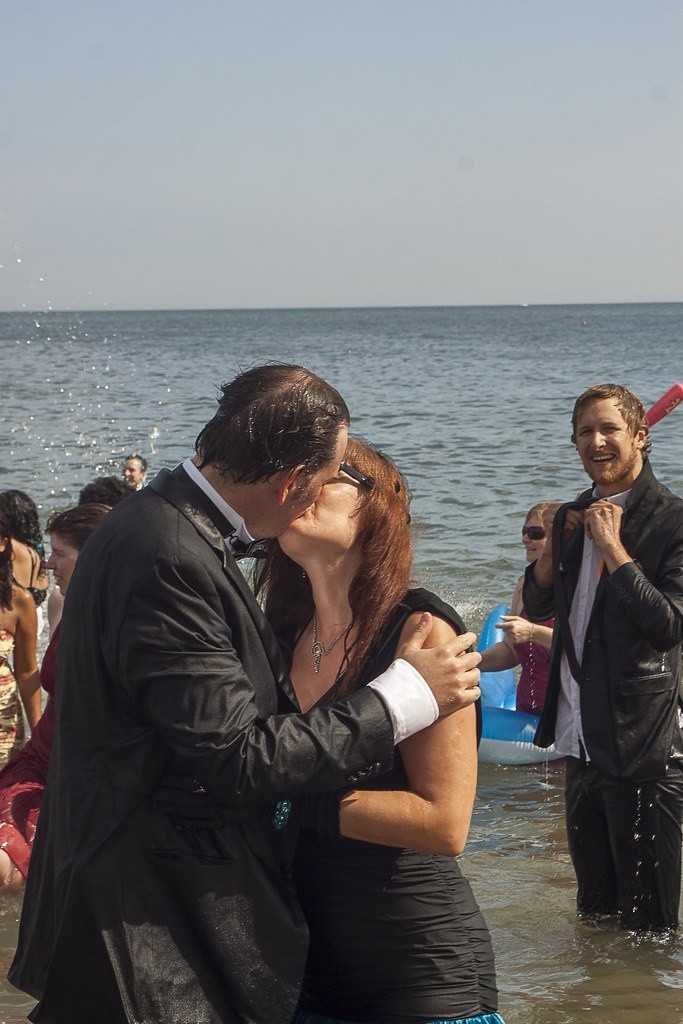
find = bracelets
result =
[530,624,534,640]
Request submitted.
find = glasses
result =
[339,462,374,491]
[522,526,546,540]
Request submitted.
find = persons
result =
[0,503,112,886]
[522,383,683,928]
[250,439,498,1024]
[79,476,136,508]
[476,502,552,716]
[7,358,480,1024]
[0,490,49,636]
[122,455,149,490]
[0,489,42,770]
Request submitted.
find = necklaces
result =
[311,618,348,674]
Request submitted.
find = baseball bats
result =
[642,383,683,428]
